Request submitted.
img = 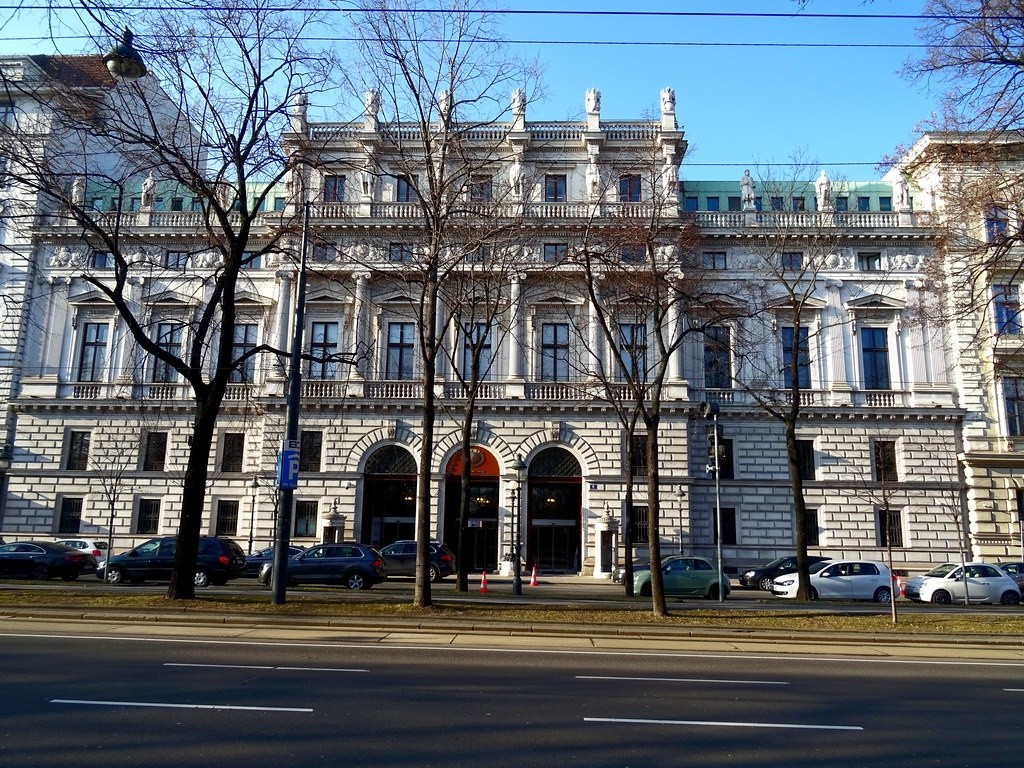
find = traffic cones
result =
[898,576,905,595]
[530,566,537,586]
[479,570,488,592]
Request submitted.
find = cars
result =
[52,539,114,563]
[991,561,1024,604]
[0,541,98,580]
[633,556,731,600]
[770,559,899,604]
[905,562,1021,605]
[239,545,315,579]
[610,554,685,585]
[738,556,833,591]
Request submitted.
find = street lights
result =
[506,479,517,552]
[513,454,526,575]
[674,484,690,553]
[248,476,260,553]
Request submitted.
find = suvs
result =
[97,537,230,586]
[378,539,456,583]
[175,534,247,580]
[258,542,388,591]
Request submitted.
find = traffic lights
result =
[699,401,719,418]
